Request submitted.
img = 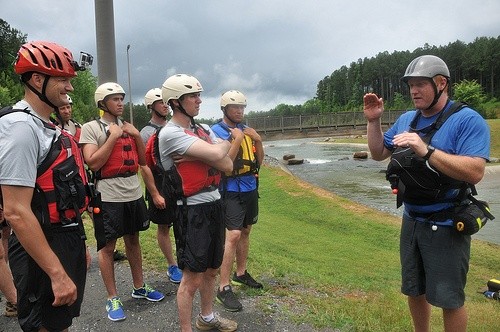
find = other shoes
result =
[5,301,17,317]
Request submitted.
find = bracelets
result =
[422,144,435,162]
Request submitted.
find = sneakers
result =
[216,284,242,312]
[113,249,128,261]
[166,264,182,283]
[105,296,126,322]
[132,282,165,302]
[196,311,238,332]
[231,269,263,290]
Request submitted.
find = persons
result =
[0,40,128,332]
[363,56,490,332]
[78,82,164,322]
[159,74,238,332]
[140,88,184,283]
[211,90,264,311]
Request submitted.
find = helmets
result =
[220,89,247,110]
[14,39,75,77]
[144,88,163,110]
[65,93,73,105]
[95,82,126,108]
[400,54,450,83]
[161,74,203,107]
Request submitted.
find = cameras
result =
[79,51,93,67]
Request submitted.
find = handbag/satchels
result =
[454,199,490,235]
